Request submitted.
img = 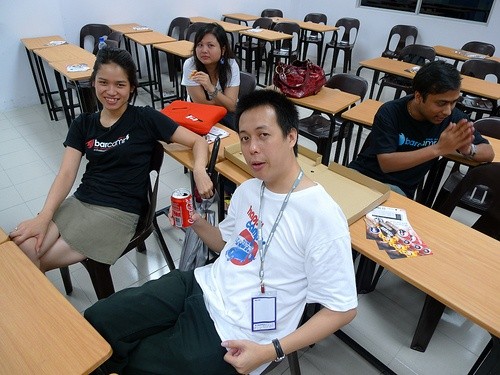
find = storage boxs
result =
[223,144,390,224]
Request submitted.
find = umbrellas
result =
[179,136,220,272]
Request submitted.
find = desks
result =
[0,13,500,375]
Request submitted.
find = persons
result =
[10,45,213,274]
[181,23,240,215]
[83,89,358,375]
[348,60,495,265]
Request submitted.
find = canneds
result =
[170,188,194,228]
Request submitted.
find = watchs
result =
[462,144,476,158]
[272,339,285,362]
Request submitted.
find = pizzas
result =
[188,70,198,79]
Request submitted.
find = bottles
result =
[98,37,107,50]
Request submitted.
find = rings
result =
[16,227,17,230]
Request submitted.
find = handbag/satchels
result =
[273,59,327,99]
[179,189,218,271]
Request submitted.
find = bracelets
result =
[208,88,218,97]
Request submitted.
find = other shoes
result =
[200,191,217,205]
[224,192,231,213]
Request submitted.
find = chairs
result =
[322,18,360,73]
[374,25,418,83]
[151,17,190,82]
[265,23,300,85]
[261,9,283,18]
[298,73,369,165]
[301,14,327,66]
[376,45,435,101]
[60,141,176,300]
[65,23,111,117]
[459,59,500,121]
[235,18,272,73]
[454,41,495,67]
[433,117,500,241]
